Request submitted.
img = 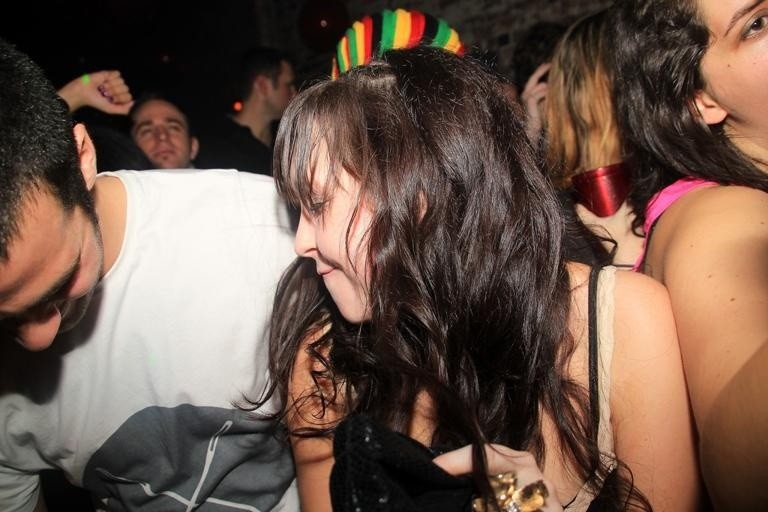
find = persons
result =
[51,38,294,174]
[270,48,694,511]
[495,6,652,272]
[620,1,766,511]
[1,52,308,512]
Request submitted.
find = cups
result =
[572,157,634,223]
